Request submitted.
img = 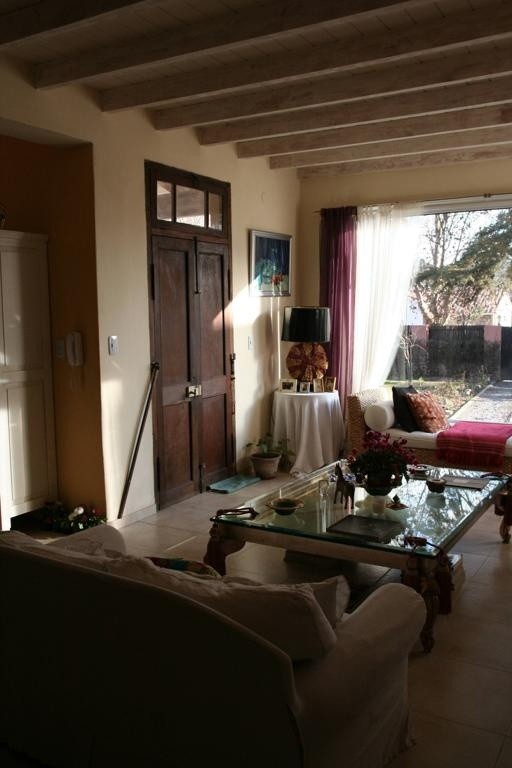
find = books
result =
[326,514,401,543]
[439,474,490,490]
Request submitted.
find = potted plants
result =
[246,432,282,479]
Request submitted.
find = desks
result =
[274,387,339,464]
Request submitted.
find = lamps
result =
[280,305,332,393]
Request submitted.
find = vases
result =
[364,474,394,496]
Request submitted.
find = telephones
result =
[66,331,83,367]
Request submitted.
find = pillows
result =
[106,556,342,664]
[222,574,351,632]
[401,390,446,433]
[392,385,419,432]
[144,556,222,581]
[364,401,395,432]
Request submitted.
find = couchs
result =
[343,384,512,474]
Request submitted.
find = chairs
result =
[0,522,428,767]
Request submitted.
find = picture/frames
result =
[248,229,293,297]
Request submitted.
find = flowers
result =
[44,503,108,532]
[347,430,421,487]
